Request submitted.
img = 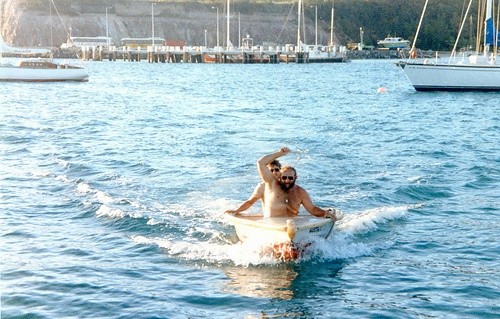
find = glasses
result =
[270,168,279,173]
[282,175,293,181]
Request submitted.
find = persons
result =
[224,160,281,216]
[257,147,333,219]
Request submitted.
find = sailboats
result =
[0,0,348,60]
[392,0,500,91]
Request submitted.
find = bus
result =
[120,38,164,48]
[67,36,112,47]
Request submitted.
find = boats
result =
[377,36,411,48]
[224,208,345,261]
[0,57,90,81]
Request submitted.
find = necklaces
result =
[285,198,288,203]
